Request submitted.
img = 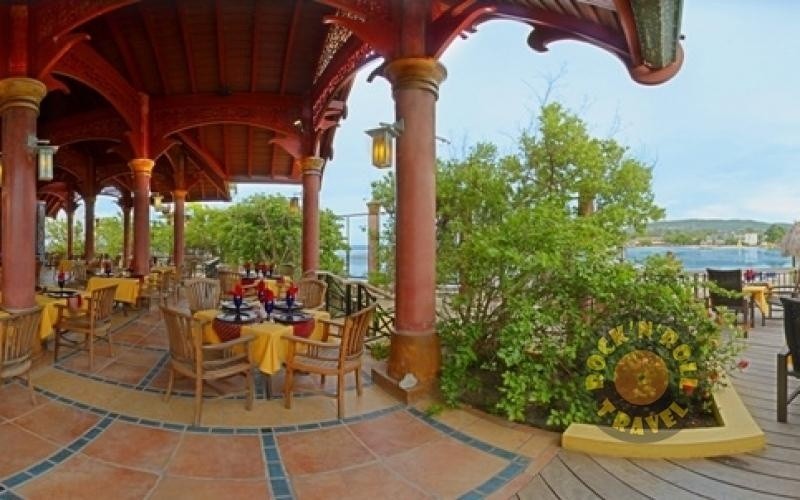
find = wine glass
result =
[264,302,273,320]
[233,296,242,311]
[286,297,293,311]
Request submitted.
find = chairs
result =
[2,250,378,428]
[705,268,800,424]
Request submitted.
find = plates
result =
[273,299,304,310]
[47,290,78,298]
[273,311,310,323]
[216,309,257,323]
[220,298,254,310]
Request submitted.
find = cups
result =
[58,280,65,291]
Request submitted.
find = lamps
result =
[365,120,405,168]
[35,138,59,182]
